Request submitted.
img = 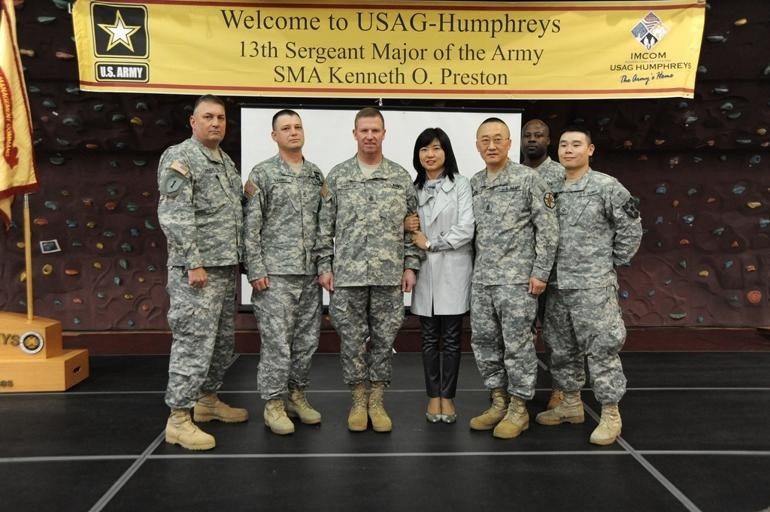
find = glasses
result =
[477,135,510,146]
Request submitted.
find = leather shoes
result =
[442,413,458,424]
[426,412,441,424]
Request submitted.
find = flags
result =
[0,0,40,231]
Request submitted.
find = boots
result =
[193,392,249,423]
[469,386,622,445]
[367,382,392,432]
[165,408,215,449]
[264,399,296,435]
[285,389,322,425]
[348,384,369,432]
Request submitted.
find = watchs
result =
[425,240,431,251]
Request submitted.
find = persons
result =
[468,117,559,440]
[240,108,326,436]
[404,126,475,425]
[519,119,563,411]
[533,112,644,445]
[155,93,251,452]
[312,105,428,434]
